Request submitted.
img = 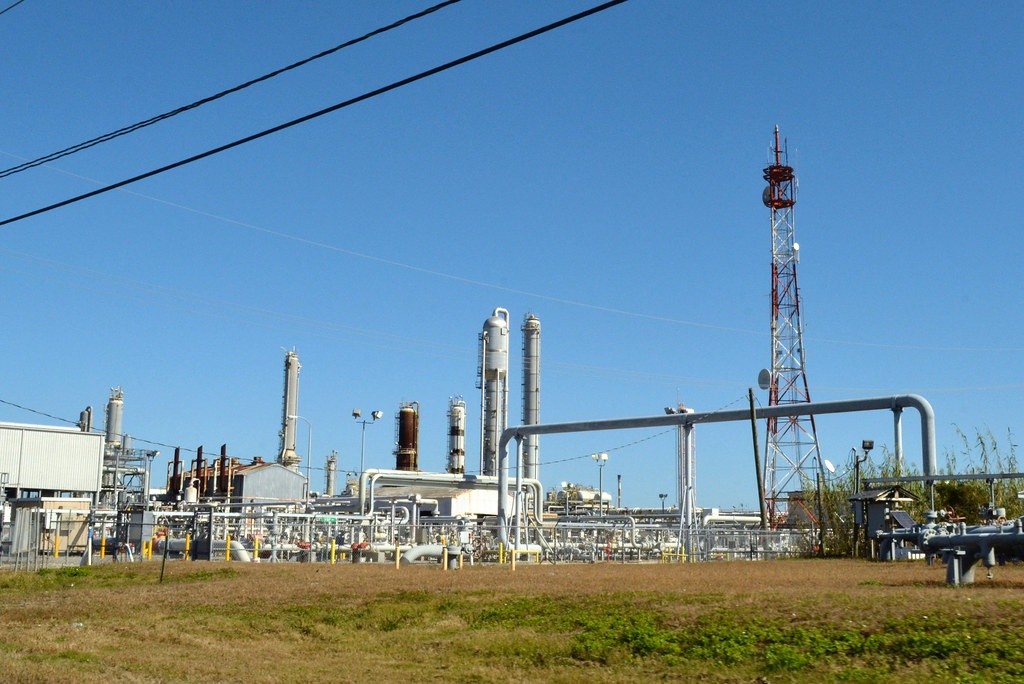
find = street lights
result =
[288,415,312,502]
[352,408,383,474]
[592,454,608,516]
[659,494,668,513]
[561,481,573,515]
[855,440,875,494]
